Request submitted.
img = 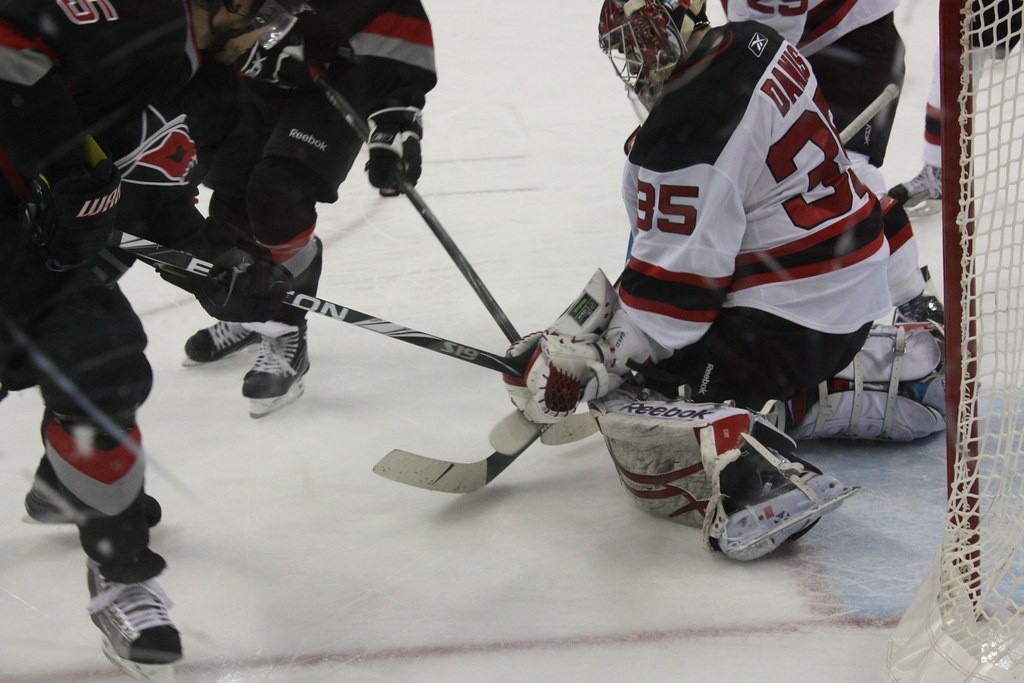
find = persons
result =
[522,1,1023,560]
[0,2,436,683]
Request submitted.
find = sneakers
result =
[238,321,324,415]
[181,321,261,365]
[892,294,945,349]
[887,163,942,218]
[25,455,162,529]
[706,438,844,562]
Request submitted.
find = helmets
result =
[248,1,319,52]
[597,0,735,85]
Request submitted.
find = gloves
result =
[365,107,423,196]
[154,216,294,323]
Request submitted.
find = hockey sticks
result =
[320,75,599,446]
[371,82,901,494]
[117,228,534,382]
